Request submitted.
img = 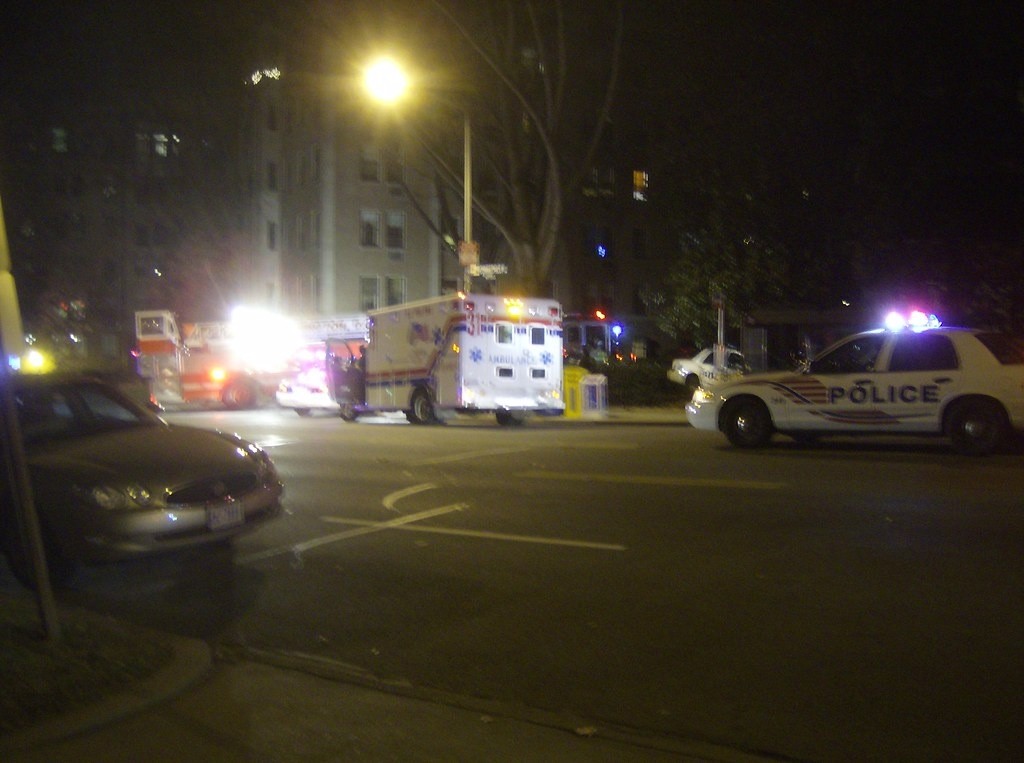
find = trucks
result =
[131,306,297,413]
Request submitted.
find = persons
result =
[788,338,808,374]
[563,342,627,408]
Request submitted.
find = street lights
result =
[370,59,473,270]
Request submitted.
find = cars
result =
[0,370,285,593]
[683,305,1024,457]
[667,342,752,398]
[275,361,343,420]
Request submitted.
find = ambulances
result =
[324,289,566,426]
